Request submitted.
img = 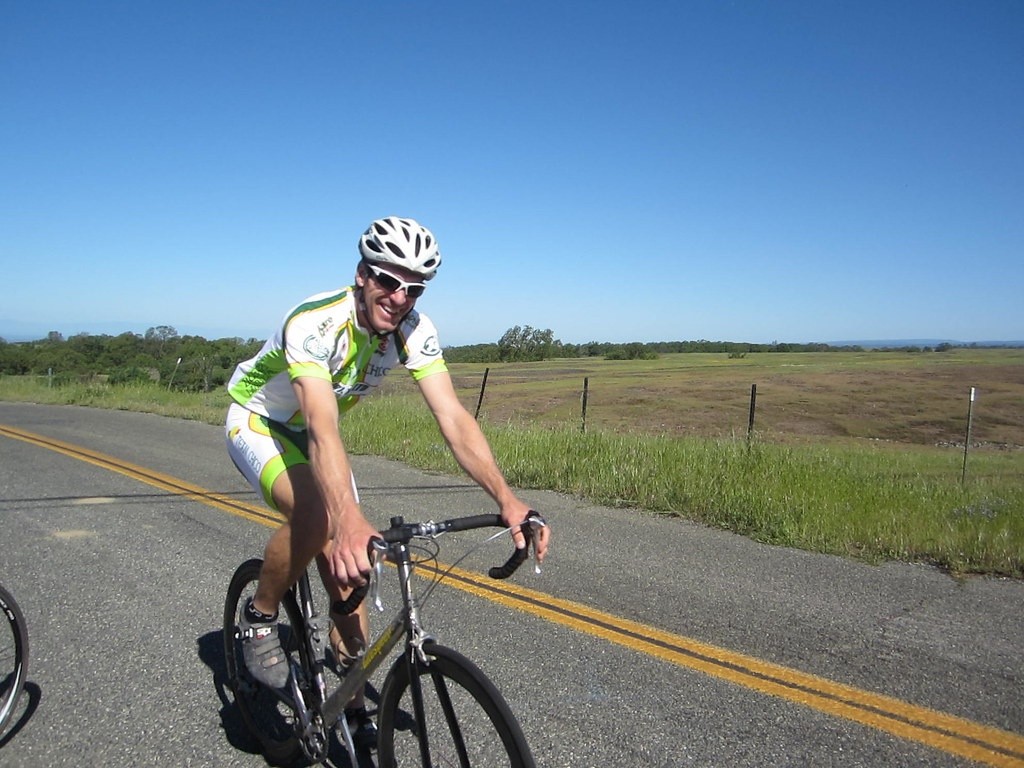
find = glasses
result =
[365,261,426,298]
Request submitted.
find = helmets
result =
[360,216,441,279]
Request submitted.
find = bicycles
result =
[222,508,547,768]
[0,585,31,737]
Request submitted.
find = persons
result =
[225,217,552,687]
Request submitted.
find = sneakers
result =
[339,711,378,752]
[235,595,290,689]
